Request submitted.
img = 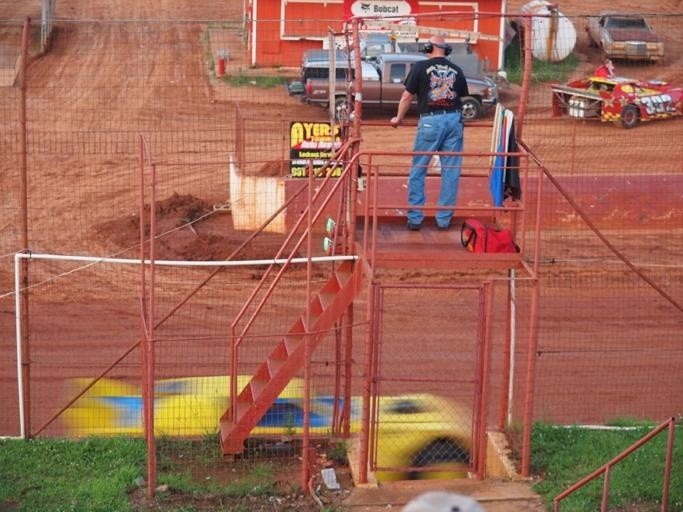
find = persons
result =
[593,59,615,78]
[388,36,469,231]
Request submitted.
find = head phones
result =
[423,42,452,56]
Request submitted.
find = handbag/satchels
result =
[460,217,516,254]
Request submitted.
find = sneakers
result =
[407,220,450,231]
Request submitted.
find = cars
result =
[584,11,664,64]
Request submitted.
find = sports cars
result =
[552,73,683,129]
[62,374,475,482]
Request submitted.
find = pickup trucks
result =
[299,33,498,121]
